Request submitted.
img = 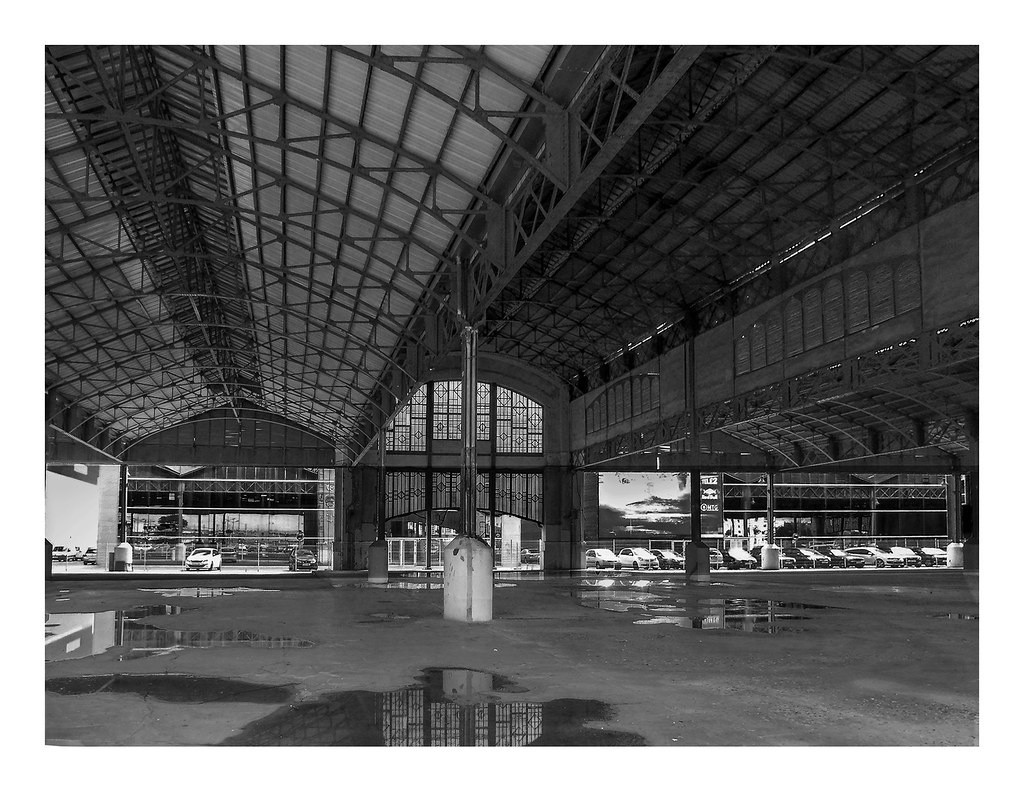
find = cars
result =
[649,549,687,571]
[910,548,949,567]
[53,545,87,562]
[288,549,319,570]
[722,548,759,568]
[782,548,832,568]
[585,549,622,570]
[521,548,540,563]
[843,546,904,568]
[877,546,921,566]
[184,548,222,571]
[83,547,98,565]
[617,548,661,570]
[219,547,238,563]
[238,544,298,553]
[682,548,724,569]
[817,549,865,568]
[749,546,796,568]
[134,543,194,555]
